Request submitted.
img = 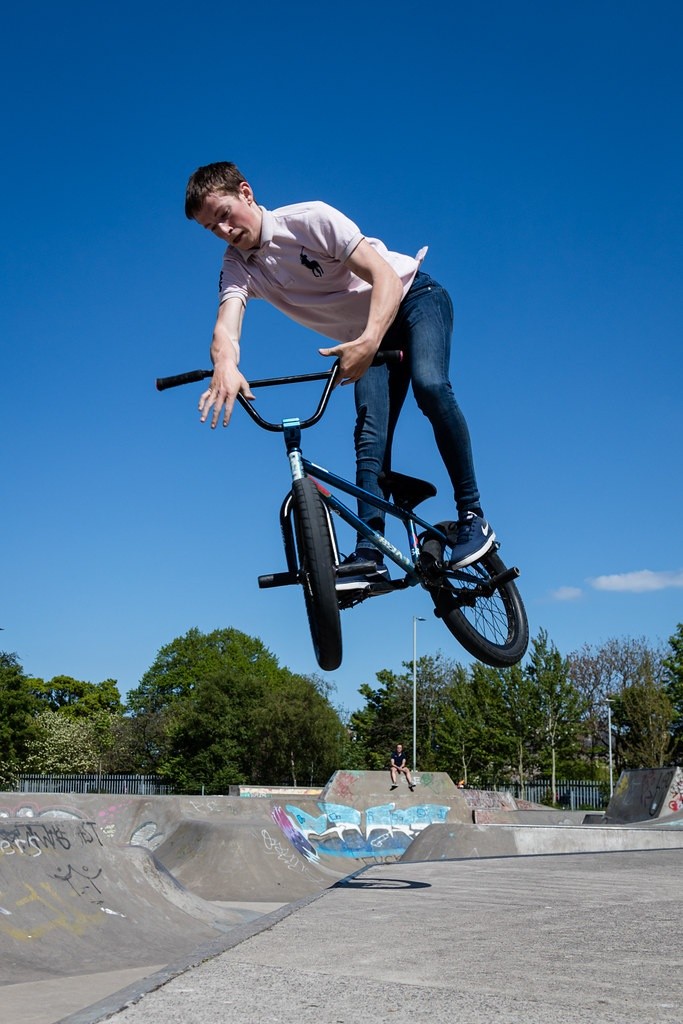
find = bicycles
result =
[155,348,531,671]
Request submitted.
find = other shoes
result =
[409,783,416,788]
[391,784,398,787]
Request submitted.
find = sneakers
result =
[333,552,391,590]
[448,512,495,570]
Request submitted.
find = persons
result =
[185,161,495,592]
[389,743,416,788]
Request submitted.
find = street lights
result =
[412,615,427,771]
[604,698,616,798]
